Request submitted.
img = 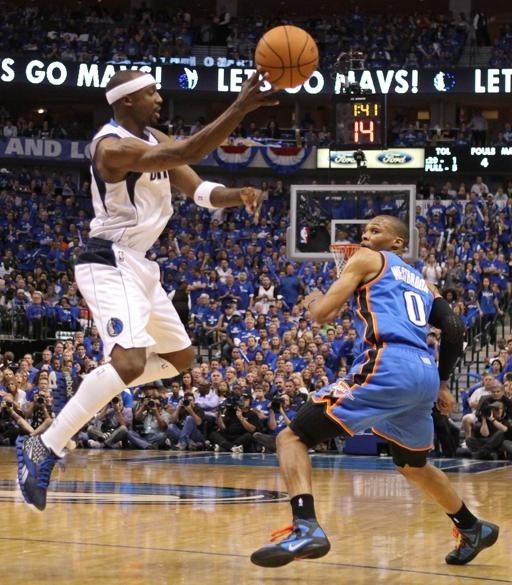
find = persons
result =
[1,1,512,460]
[15,65,279,515]
[251,215,499,567]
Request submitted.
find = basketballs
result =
[256,25,317,89]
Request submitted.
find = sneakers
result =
[88,439,101,449]
[250,518,330,568]
[15,435,62,513]
[171,440,245,453]
[444,519,498,565]
